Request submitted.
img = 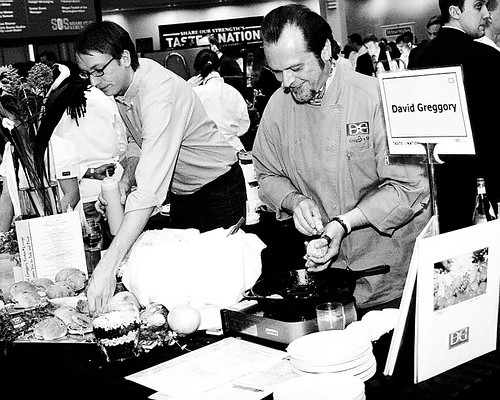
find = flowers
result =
[0,61,92,186]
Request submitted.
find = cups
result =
[82,219,103,251]
[316,303,346,332]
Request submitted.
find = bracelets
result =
[327,216,351,237]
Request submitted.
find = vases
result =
[18,180,62,218]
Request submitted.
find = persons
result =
[73,20,247,317]
[0,0,500,234]
[251,3,432,400]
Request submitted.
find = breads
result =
[10,268,85,340]
[307,239,328,258]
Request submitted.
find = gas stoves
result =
[220,296,358,344]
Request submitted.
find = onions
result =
[167,302,201,335]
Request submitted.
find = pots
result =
[242,264,390,315]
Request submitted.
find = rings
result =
[322,235,331,245]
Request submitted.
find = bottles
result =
[471,178,497,225]
[101,171,124,235]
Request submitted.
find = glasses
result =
[79,57,115,80]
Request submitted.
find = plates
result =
[273,373,366,400]
[287,330,377,382]
